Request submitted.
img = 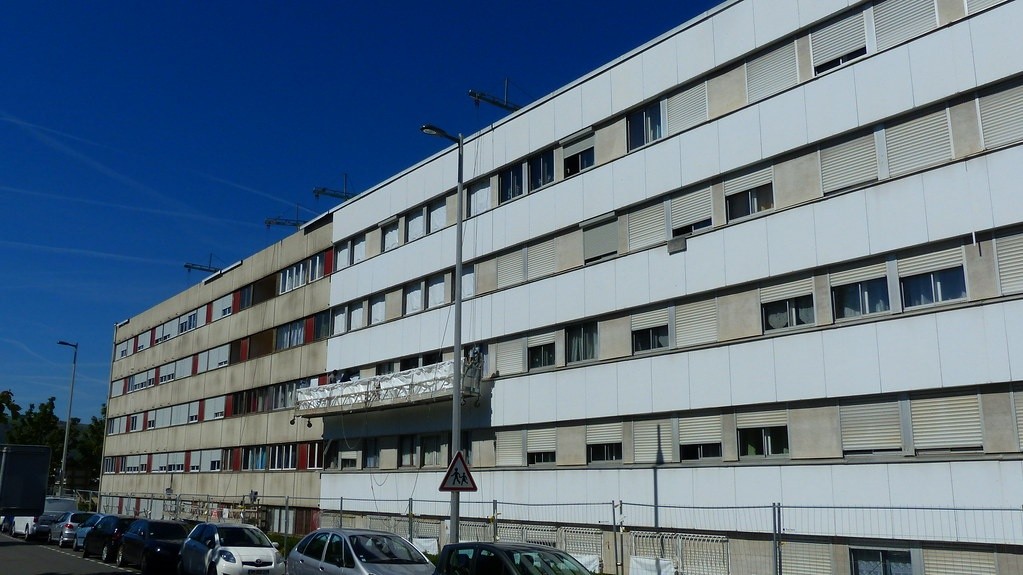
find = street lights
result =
[57,341,78,497]
[420,122,463,542]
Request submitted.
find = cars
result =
[32,511,61,542]
[82,515,141,563]
[177,521,286,575]
[0,516,11,532]
[287,527,436,575]
[432,541,592,575]
[11,516,39,540]
[73,513,111,552]
[116,519,190,573]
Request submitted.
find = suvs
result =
[48,510,98,548]
